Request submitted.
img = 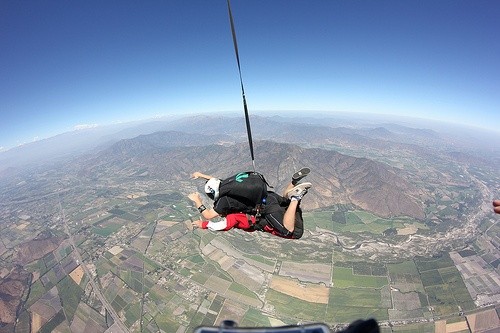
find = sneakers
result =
[286,182,312,200]
[292,167,311,182]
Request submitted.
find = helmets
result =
[204,178,222,201]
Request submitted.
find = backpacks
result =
[213,171,273,216]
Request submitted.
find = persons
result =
[493,200,500,214]
[188,167,312,239]
